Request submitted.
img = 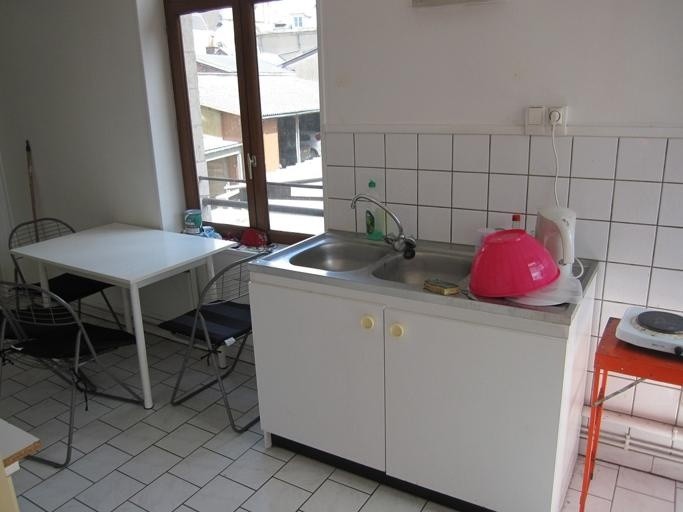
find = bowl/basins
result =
[468,229,560,297]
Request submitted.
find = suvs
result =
[279,130,321,158]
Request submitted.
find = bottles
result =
[512,213,520,229]
[183,209,202,236]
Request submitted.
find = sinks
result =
[372,249,473,291]
[262,229,392,272]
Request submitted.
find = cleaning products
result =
[364,177,384,238]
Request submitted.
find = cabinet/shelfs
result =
[246,281,599,511]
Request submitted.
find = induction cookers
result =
[615,306,683,354]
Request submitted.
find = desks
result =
[7,220,231,410]
[579,317,683,511]
[0,419,44,512]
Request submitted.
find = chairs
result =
[154,250,271,435]
[7,215,127,334]
[1,278,145,469]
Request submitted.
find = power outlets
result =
[546,105,569,137]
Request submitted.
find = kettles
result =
[535,206,577,275]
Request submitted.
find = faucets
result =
[350,193,404,240]
